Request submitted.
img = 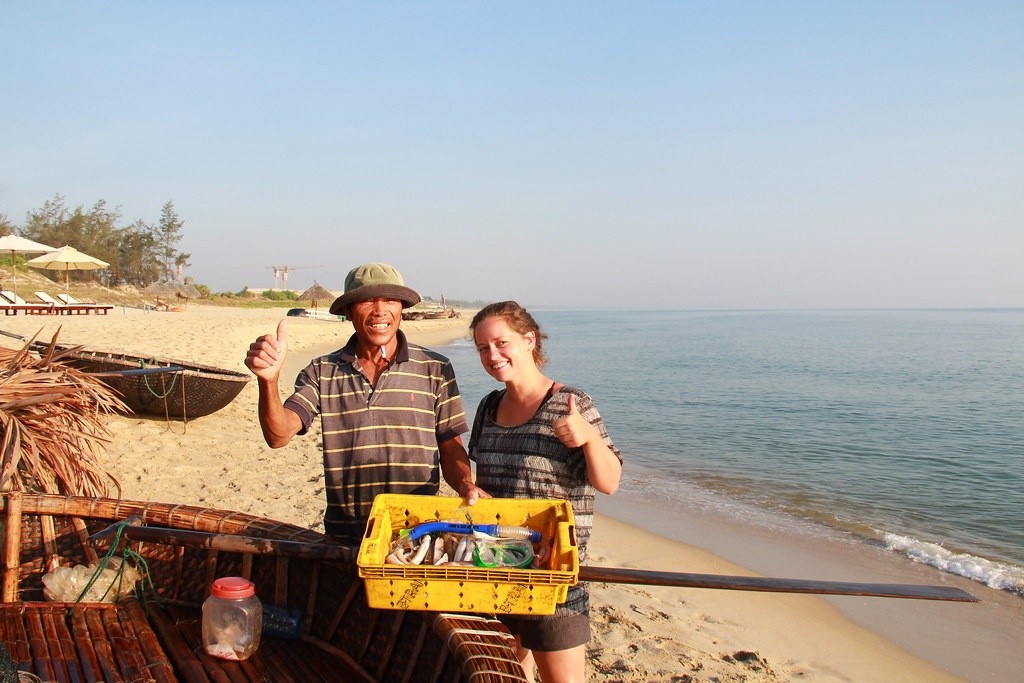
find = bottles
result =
[201,576,263,661]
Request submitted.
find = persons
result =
[467,300,623,683]
[244,263,492,547]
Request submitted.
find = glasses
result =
[471,536,534,568]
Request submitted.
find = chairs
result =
[0,290,52,315]
[34,291,113,315]
[57,293,96,315]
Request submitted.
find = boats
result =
[402,312,425,320]
[421,311,451,318]
[0,493,525,683]
[28,341,250,419]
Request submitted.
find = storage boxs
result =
[354,493,579,616]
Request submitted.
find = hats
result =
[329,263,421,316]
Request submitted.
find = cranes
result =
[265,265,280,290]
[280,264,325,290]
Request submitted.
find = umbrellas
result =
[146,281,202,310]
[24,246,110,305]
[0,235,60,304]
[295,280,337,308]
